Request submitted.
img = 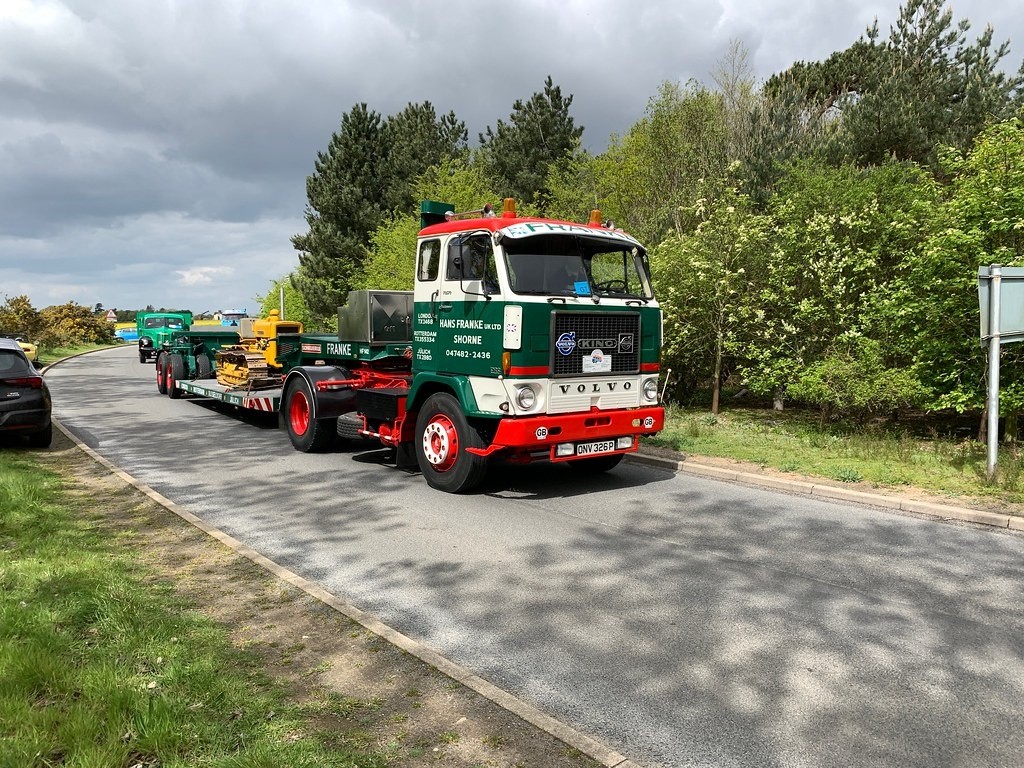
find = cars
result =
[0,338,53,447]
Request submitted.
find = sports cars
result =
[0,333,40,362]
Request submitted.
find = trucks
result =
[137,311,193,362]
[152,200,672,495]
